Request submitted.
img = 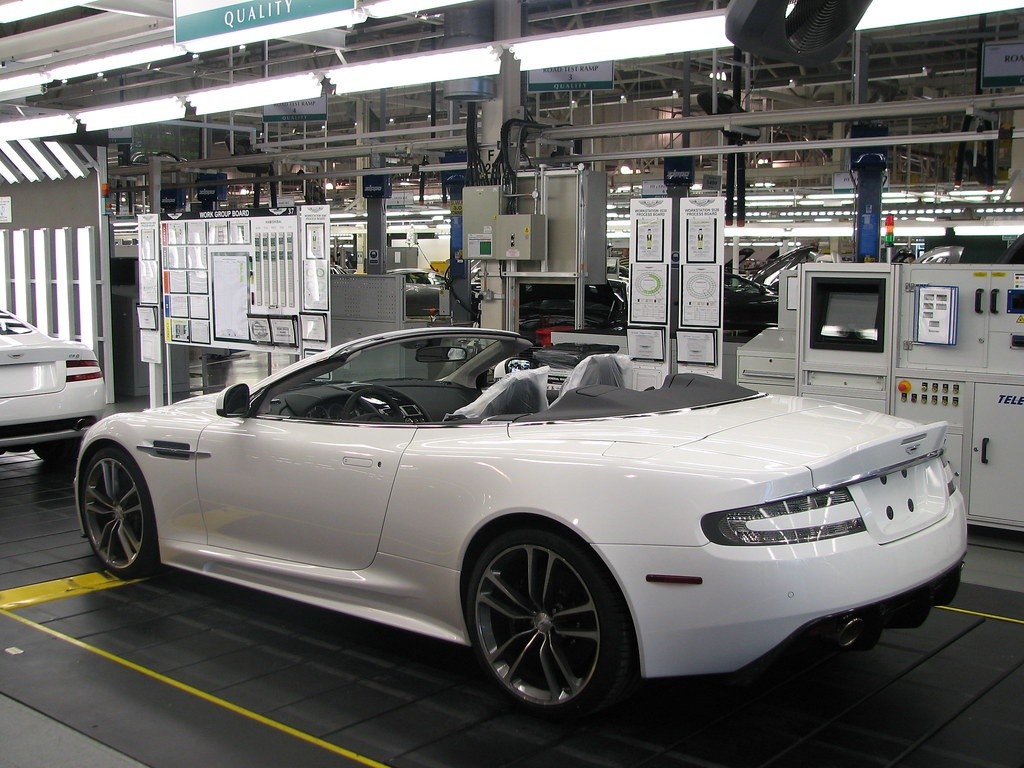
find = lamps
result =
[18,139,67,181]
[77,225,99,363]
[0,229,12,313]
[0,140,45,182]
[55,227,75,341]
[0,0,1023,141]
[328,220,1024,237]
[45,142,90,179]
[34,227,54,338]
[0,149,24,184]
[12,228,32,325]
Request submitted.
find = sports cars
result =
[73,323,968,722]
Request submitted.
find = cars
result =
[519,234,1024,347]
[385,267,482,325]
[0,307,108,465]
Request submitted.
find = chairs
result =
[444,365,549,423]
[417,276,431,284]
[559,354,633,398]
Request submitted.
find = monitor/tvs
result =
[825,292,878,339]
